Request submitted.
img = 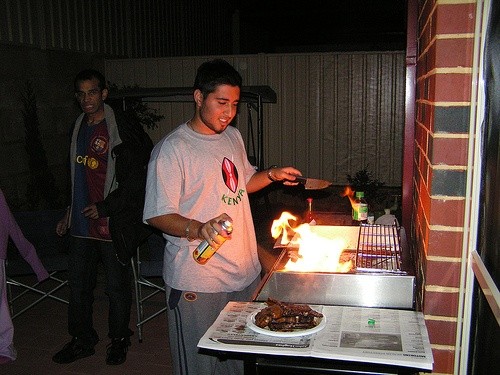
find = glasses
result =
[75,88,101,100]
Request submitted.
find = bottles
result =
[193,220,233,265]
[305,197,316,222]
[351,192,370,226]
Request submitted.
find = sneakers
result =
[106,335,130,365]
[52,340,95,364]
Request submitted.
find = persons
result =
[141,59,302,375]
[49,72,155,365]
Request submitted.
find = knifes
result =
[286,176,333,190]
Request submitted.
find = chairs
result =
[131,242,167,341]
[5,220,70,320]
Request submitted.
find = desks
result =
[198,301,434,375]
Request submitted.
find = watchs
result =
[267,164,278,182]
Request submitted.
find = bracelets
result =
[185,220,198,242]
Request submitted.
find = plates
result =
[246,305,327,338]
